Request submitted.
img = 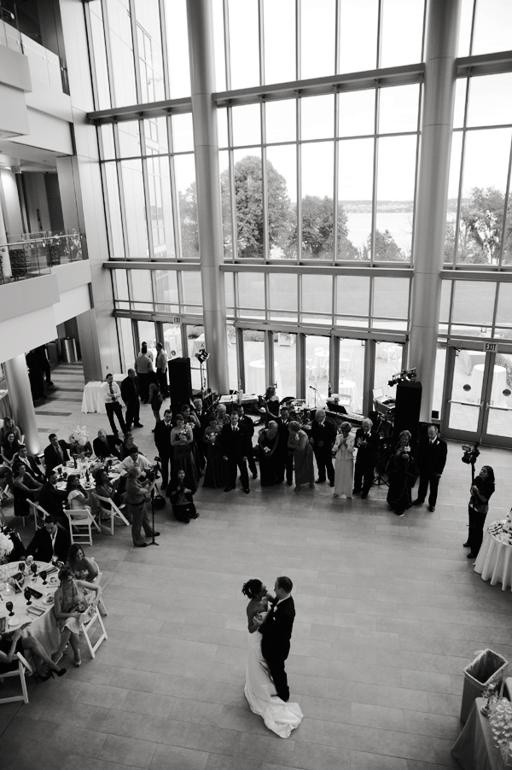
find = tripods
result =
[373,453,390,487]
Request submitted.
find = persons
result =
[20,228,89,265]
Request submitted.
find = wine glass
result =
[54,454,112,483]
[6,601,15,616]
[18,562,48,585]
[487,694,511,748]
[24,591,32,606]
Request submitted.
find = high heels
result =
[48,667,67,679]
[73,648,82,667]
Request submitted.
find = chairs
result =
[2,427,147,546]
[0,532,108,662]
[0,628,33,706]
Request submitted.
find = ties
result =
[56,447,62,460]
[108,384,116,402]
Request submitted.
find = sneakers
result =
[159,473,437,519]
[133,422,143,428]
[462,540,471,548]
[146,531,160,537]
[133,543,147,548]
[466,552,477,559]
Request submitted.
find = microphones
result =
[309,385,318,391]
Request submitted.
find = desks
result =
[472,518,511,593]
[450,694,511,770]
[82,381,122,414]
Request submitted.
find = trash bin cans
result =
[461,648,509,724]
